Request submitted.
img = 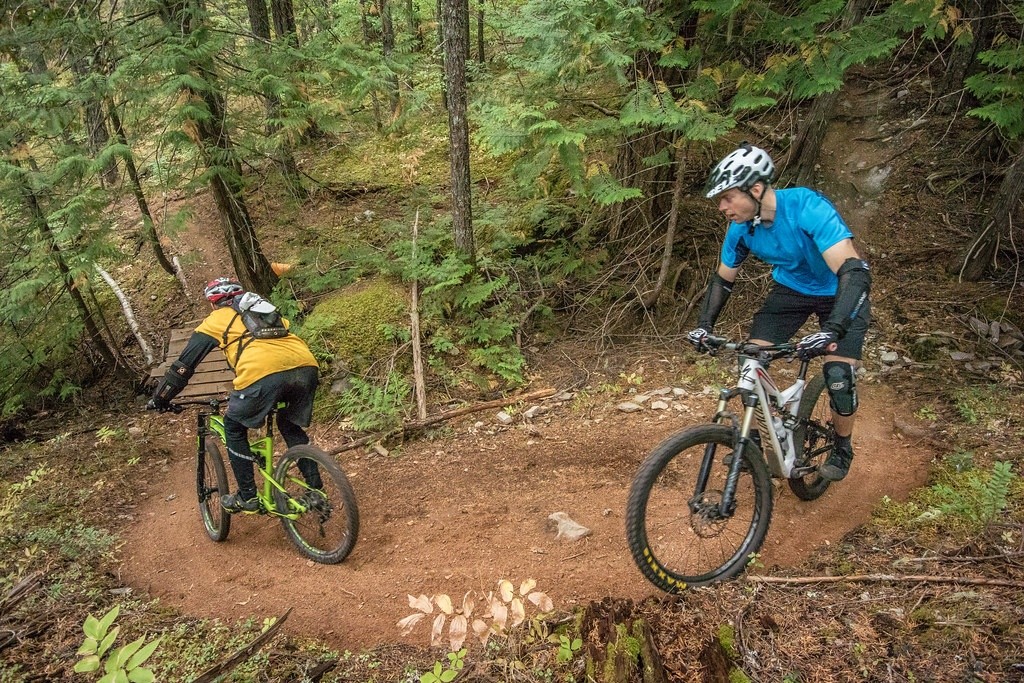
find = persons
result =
[148,277,327,515]
[687,144,873,481]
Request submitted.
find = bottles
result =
[772,416,790,452]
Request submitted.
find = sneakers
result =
[219,489,260,515]
[723,442,764,465]
[304,488,327,505]
[820,444,854,481]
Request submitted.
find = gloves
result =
[687,326,713,348]
[147,397,171,410]
[796,332,840,360]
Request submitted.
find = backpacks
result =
[231,293,286,338]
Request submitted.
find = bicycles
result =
[622,334,832,596]
[143,395,361,567]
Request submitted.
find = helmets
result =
[706,146,776,198]
[205,277,243,302]
